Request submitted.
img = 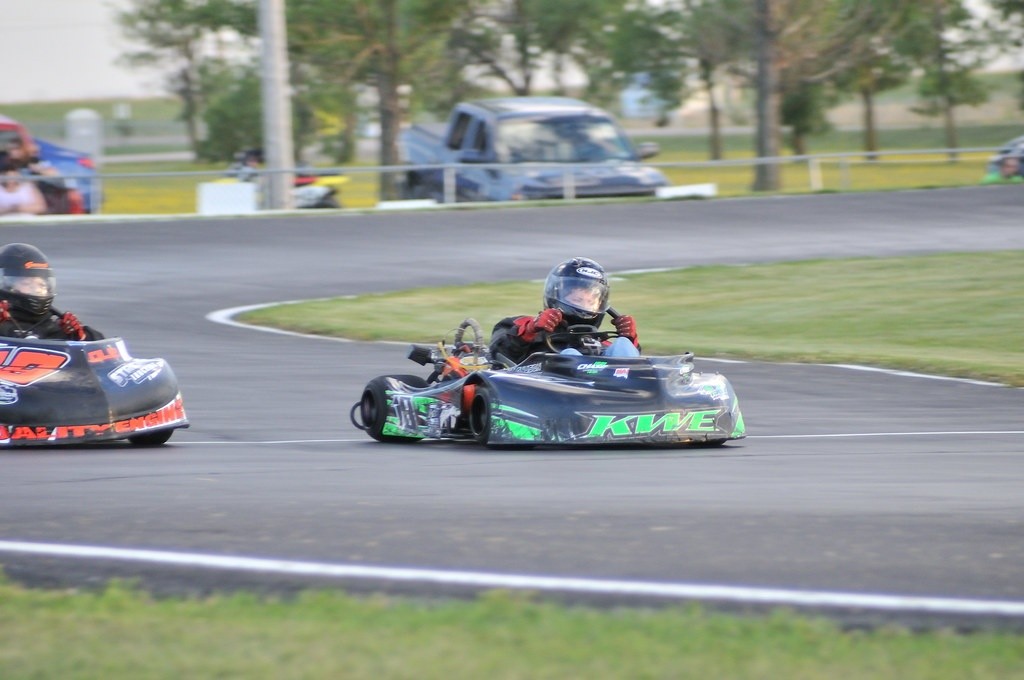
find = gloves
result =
[57,311,86,341]
[612,315,639,349]
[0,300,11,322]
[530,309,561,333]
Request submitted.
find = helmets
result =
[0,242,55,315]
[544,257,611,330]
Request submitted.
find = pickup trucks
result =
[407,98,667,204]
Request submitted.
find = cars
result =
[35,140,101,215]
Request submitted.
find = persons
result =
[489,258,642,371]
[0,243,104,342]
[0,137,86,214]
[978,157,1024,186]
